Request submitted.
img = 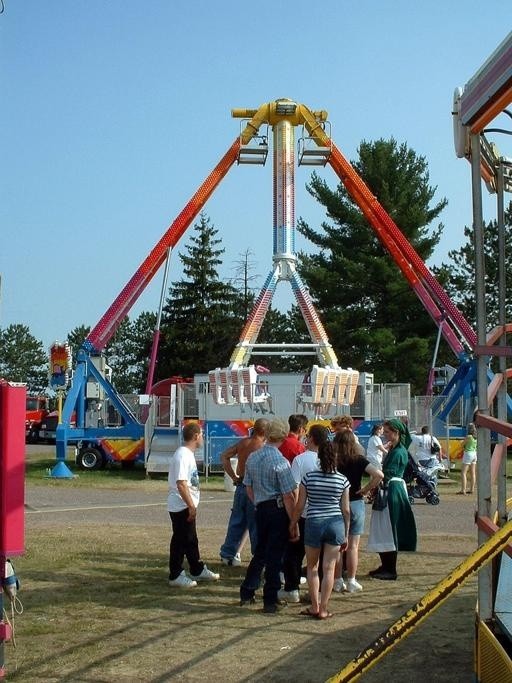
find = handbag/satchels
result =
[431,436,440,454]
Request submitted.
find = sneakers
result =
[169,569,197,588]
[303,591,322,604]
[220,556,233,567]
[241,595,262,606]
[277,588,301,603]
[280,572,307,584]
[262,599,288,614]
[346,581,363,591]
[190,563,220,582]
[333,581,346,592]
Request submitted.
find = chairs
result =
[334,367,350,404]
[218,367,238,405]
[300,365,327,403]
[231,366,249,403]
[241,365,267,403]
[321,365,338,403]
[209,367,226,405]
[345,368,359,404]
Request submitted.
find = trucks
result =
[26,397,50,444]
[39,398,77,444]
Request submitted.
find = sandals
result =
[367,493,375,501]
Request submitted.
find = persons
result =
[288,441,351,621]
[167,422,221,588]
[455,423,478,495]
[365,424,389,505]
[366,419,417,580]
[277,425,329,603]
[409,425,444,489]
[294,369,336,421]
[332,430,385,592]
[240,416,301,614]
[278,414,308,465]
[331,414,365,456]
[220,418,270,566]
[238,380,275,415]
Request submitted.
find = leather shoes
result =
[369,566,386,578]
[373,571,397,580]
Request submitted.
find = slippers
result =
[456,490,467,494]
[301,608,319,616]
[317,610,334,619]
[467,489,474,493]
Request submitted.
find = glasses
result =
[303,427,307,433]
[306,434,311,438]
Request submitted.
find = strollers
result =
[389,441,440,505]
